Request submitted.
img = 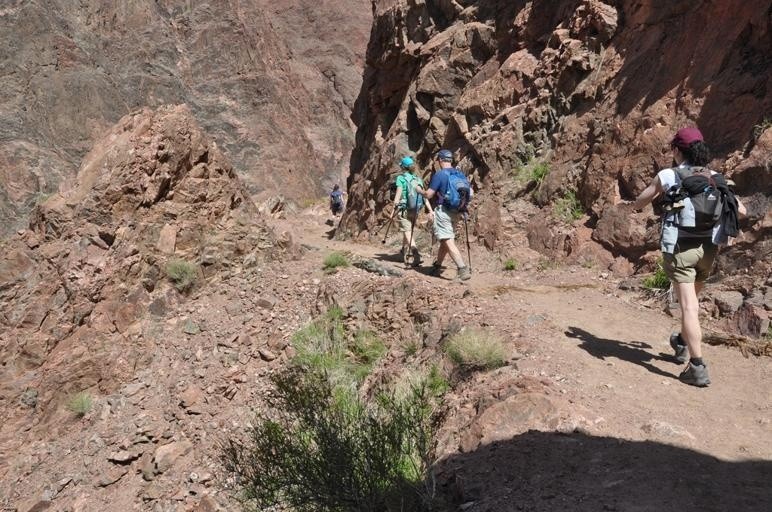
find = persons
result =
[329,183,344,218]
[391,156,434,268]
[632,125,746,387]
[416,150,472,281]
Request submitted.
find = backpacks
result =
[332,196,343,210]
[681,176,720,227]
[400,174,424,209]
[441,170,470,210]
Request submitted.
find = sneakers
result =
[670,332,690,363]
[458,266,471,281]
[403,248,419,267]
[680,362,711,386]
[425,265,439,276]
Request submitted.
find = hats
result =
[436,150,453,161]
[399,157,414,167]
[668,126,703,146]
